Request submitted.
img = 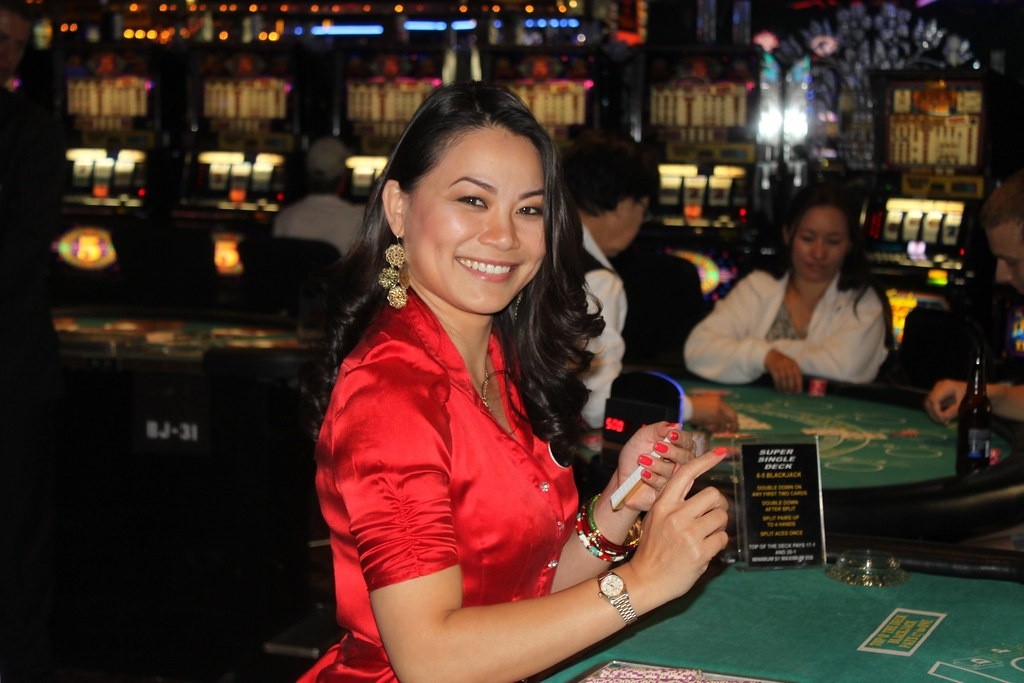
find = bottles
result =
[956,348,993,476]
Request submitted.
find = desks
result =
[64,191,275,235]
[516,538,1024,683]
[47,306,325,375]
[578,360,1024,544]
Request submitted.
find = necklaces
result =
[481,368,492,413]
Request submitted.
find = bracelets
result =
[576,493,643,562]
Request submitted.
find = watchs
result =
[596,570,638,624]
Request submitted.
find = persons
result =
[559,126,661,506]
[274,139,365,260]
[296,78,728,683]
[611,204,707,363]
[925,170,1024,426]
[684,180,896,394]
